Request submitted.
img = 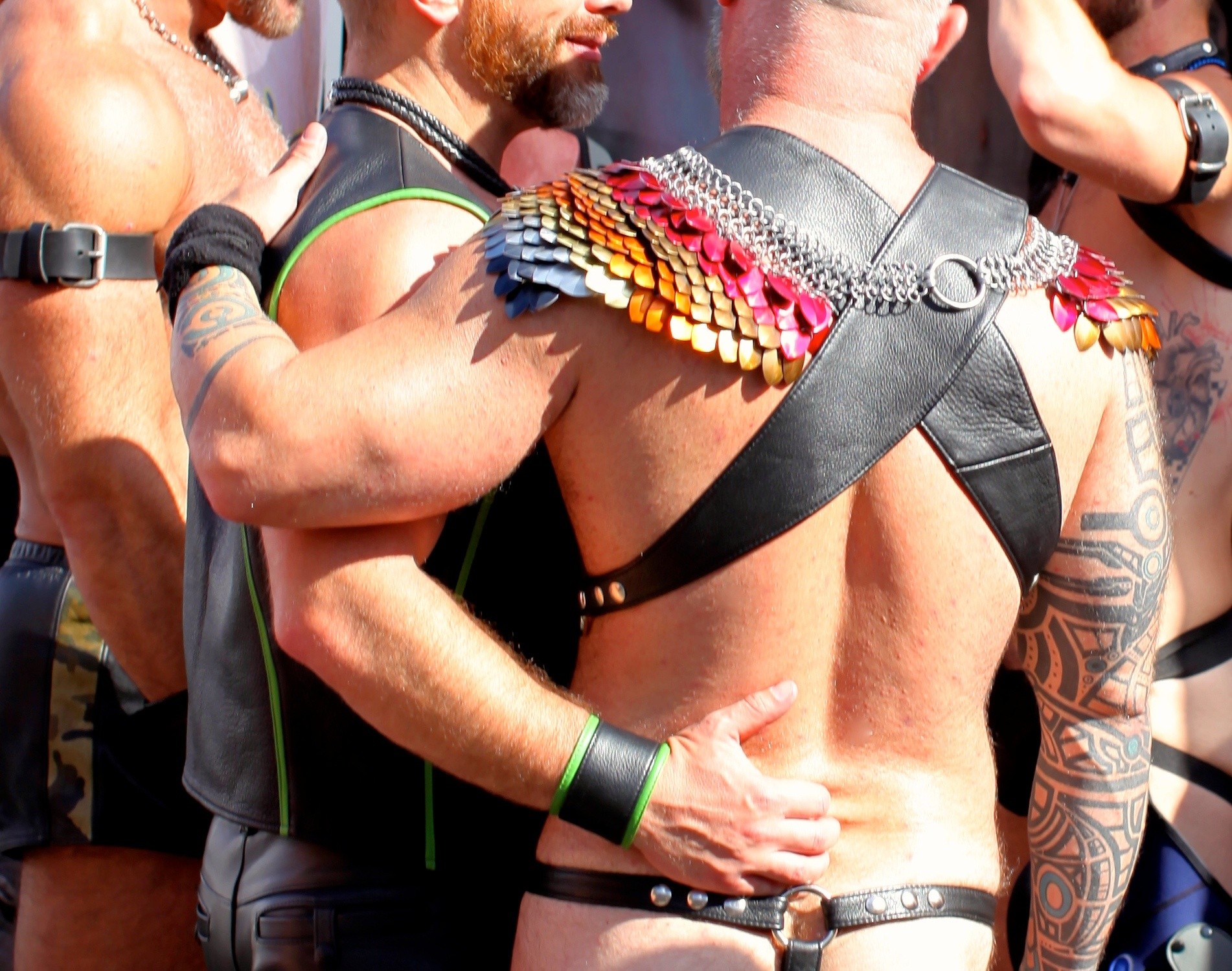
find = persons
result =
[0,0,1232,971]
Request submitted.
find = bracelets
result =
[550,715,670,850]
[161,205,265,327]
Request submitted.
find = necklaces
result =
[134,0,249,104]
[332,75,510,186]
[1050,168,1080,234]
[331,89,506,197]
